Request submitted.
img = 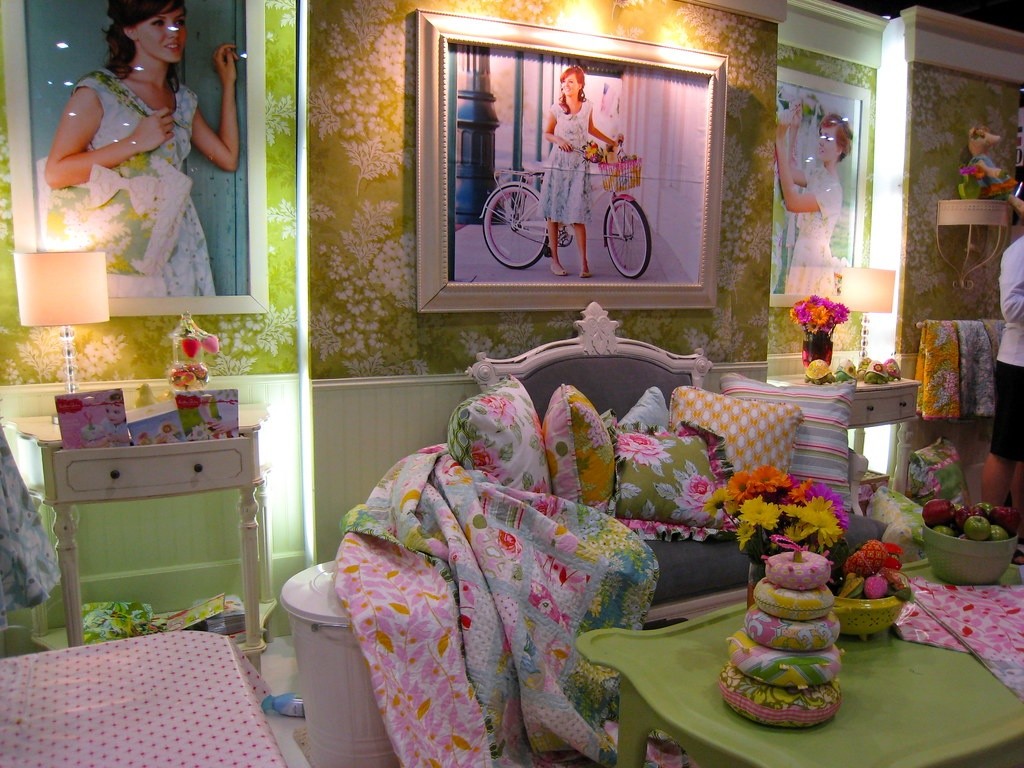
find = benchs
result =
[336,300,889,768]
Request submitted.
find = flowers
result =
[579,140,606,165]
[703,464,851,587]
[790,296,850,357]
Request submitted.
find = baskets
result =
[598,158,642,193]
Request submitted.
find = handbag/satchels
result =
[36,67,193,279]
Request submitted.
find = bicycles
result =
[478,141,653,280]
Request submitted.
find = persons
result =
[99,393,131,447]
[199,390,239,438]
[44,0,238,299]
[776,102,854,296]
[982,234,1024,565]
[536,65,624,277]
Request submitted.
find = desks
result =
[577,558,1024,768]
[0,404,278,680]
[0,632,289,768]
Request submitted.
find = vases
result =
[746,562,766,610]
[801,330,834,368]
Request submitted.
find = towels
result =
[913,318,1005,421]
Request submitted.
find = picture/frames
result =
[414,8,729,313]
[1,0,270,318]
[769,66,873,308]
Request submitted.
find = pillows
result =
[447,374,552,497]
[866,486,926,563]
[722,372,857,512]
[671,384,803,476]
[601,408,742,541]
[908,436,971,509]
[620,385,671,432]
[543,384,616,513]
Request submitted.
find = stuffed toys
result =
[968,120,1024,222]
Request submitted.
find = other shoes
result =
[579,272,592,278]
[550,265,567,275]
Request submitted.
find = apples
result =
[922,498,1020,541]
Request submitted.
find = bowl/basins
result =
[833,572,911,642]
[923,522,1019,585]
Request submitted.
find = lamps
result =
[839,267,896,455]
[12,251,109,426]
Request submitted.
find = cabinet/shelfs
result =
[766,375,923,496]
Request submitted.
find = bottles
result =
[166,311,209,391]
